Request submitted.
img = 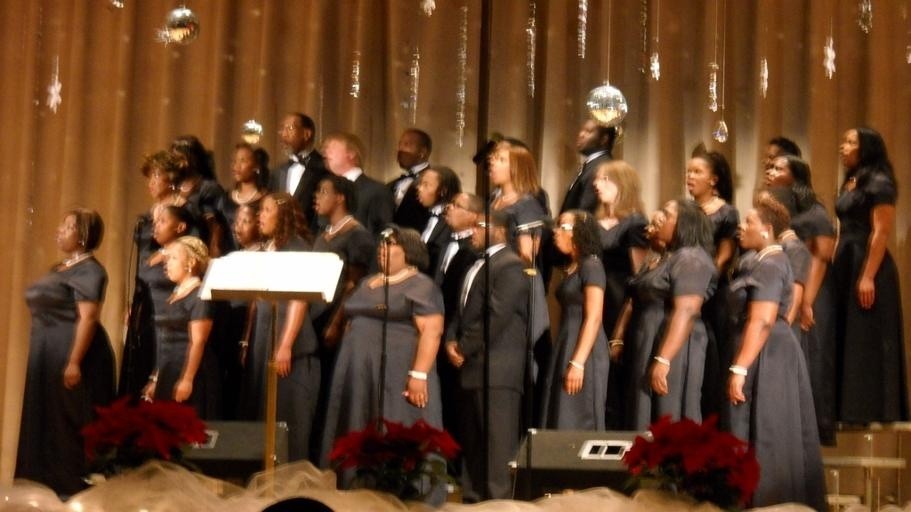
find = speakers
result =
[182,421,289,480]
[508,427,653,500]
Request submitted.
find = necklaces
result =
[65,250,94,267]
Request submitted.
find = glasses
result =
[556,224,573,231]
[279,124,298,133]
[449,200,474,215]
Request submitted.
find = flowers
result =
[621,413,762,511]
[325,415,461,498]
[79,394,209,467]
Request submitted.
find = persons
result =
[13,208,117,503]
[116,110,841,512]
[832,126,905,430]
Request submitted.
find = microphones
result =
[473,140,495,161]
[380,227,394,240]
[513,219,546,236]
[134,215,154,232]
